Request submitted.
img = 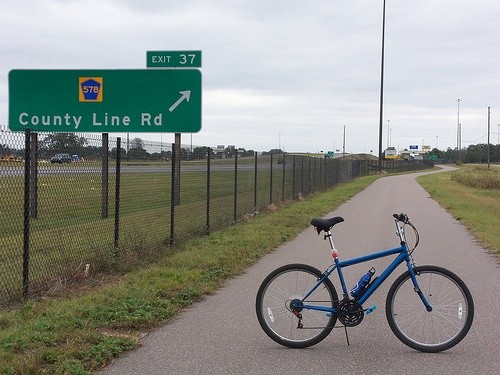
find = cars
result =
[278,158,286,164]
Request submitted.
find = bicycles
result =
[255,213,474,353]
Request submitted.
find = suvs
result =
[51,153,71,164]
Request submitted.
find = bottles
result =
[350,267,376,298]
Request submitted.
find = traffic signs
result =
[7,68,202,136]
